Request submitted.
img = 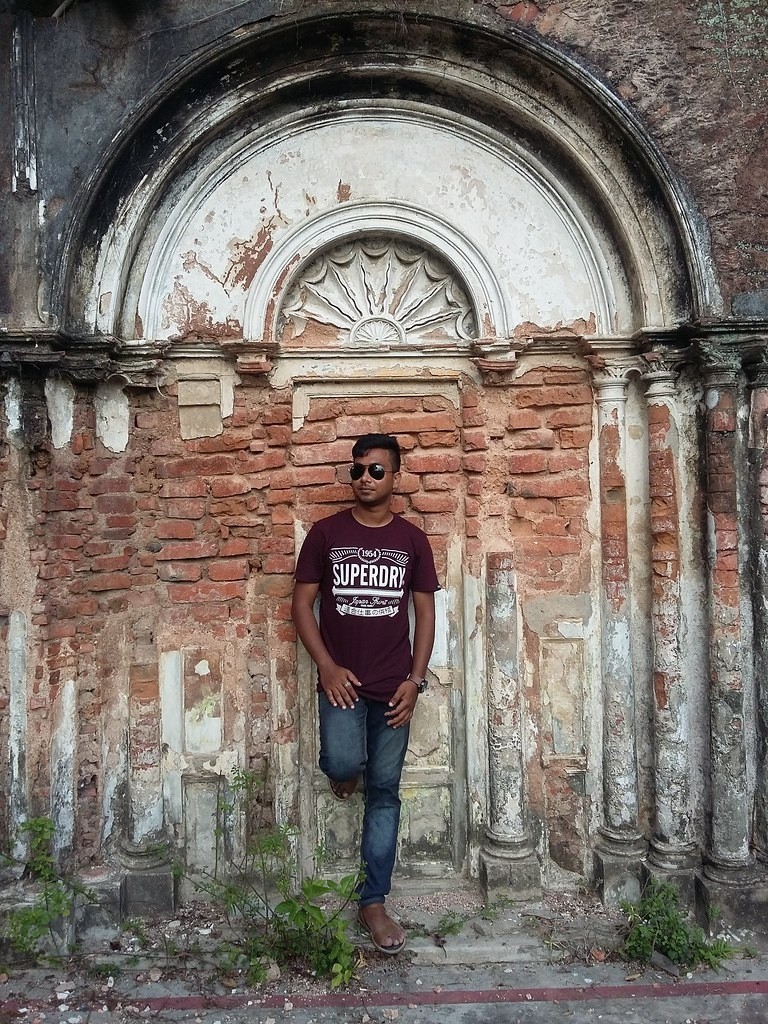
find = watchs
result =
[406,673,429,693]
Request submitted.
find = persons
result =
[290,432,437,954]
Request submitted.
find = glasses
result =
[350,463,396,480]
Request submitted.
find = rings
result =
[343,681,351,688]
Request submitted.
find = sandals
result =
[328,776,357,802]
[357,906,407,954]
